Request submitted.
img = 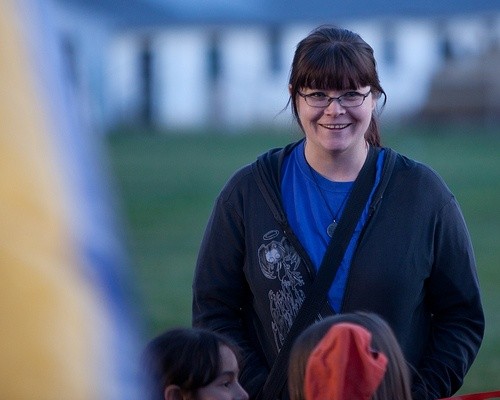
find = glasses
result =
[297,89,371,107]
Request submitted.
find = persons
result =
[143,328,254,398]
[189,26,487,400]
[283,310,414,400]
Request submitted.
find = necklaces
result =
[303,137,355,242]
